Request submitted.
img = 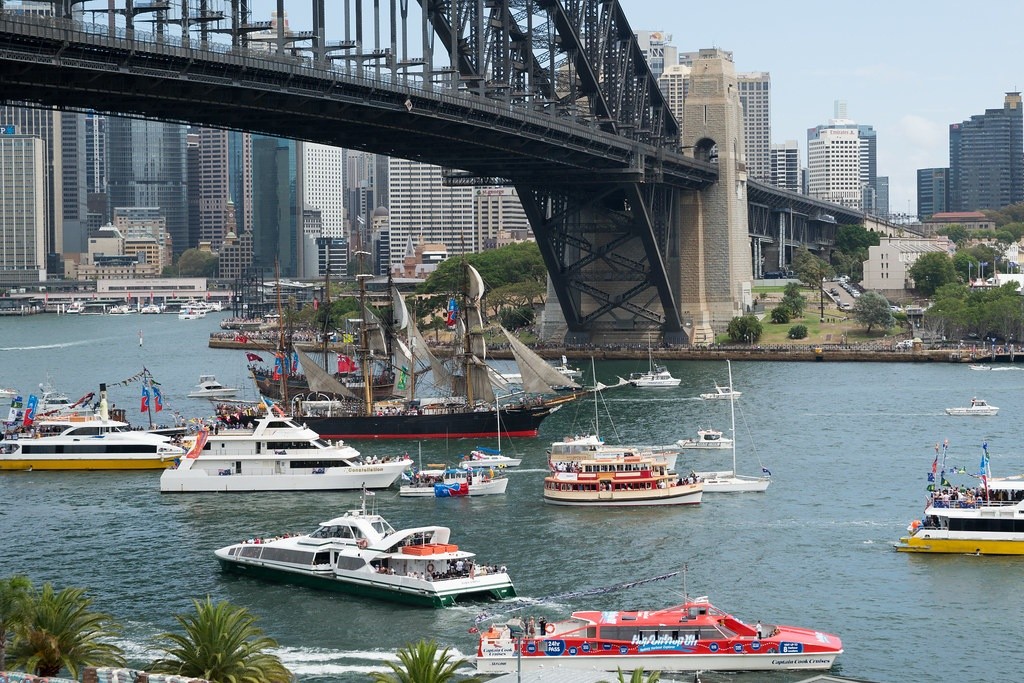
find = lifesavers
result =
[427,563,434,572]
[499,464,503,468]
[545,623,555,633]
[363,540,367,548]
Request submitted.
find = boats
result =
[700,379,742,399]
[161,397,414,493]
[67,300,223,320]
[213,482,518,608]
[489,367,586,392]
[542,454,704,506]
[894,439,1024,556]
[475,563,844,675]
[945,400,1000,416]
[0,365,187,471]
[675,426,733,449]
[969,366,992,370]
[188,375,238,397]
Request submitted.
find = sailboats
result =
[208,217,589,441]
[245,261,453,403]
[628,333,682,387]
[547,355,679,471]
[693,359,771,493]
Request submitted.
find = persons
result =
[303,410,325,417]
[372,394,542,418]
[922,484,1024,530]
[213,330,311,341]
[507,615,547,639]
[375,532,499,580]
[173,433,181,443]
[0,447,4,454]
[360,450,505,489]
[219,469,231,476]
[312,468,324,474]
[755,620,777,640]
[554,460,701,492]
[131,425,168,431]
[189,402,259,436]
[6,425,60,440]
[240,531,302,544]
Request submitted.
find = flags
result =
[447,298,459,312]
[343,334,354,343]
[338,360,356,372]
[314,299,318,312]
[334,352,358,372]
[273,351,299,381]
[246,352,265,362]
[446,311,457,326]
[141,381,162,412]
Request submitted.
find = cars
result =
[891,306,899,311]
[823,274,860,306]
